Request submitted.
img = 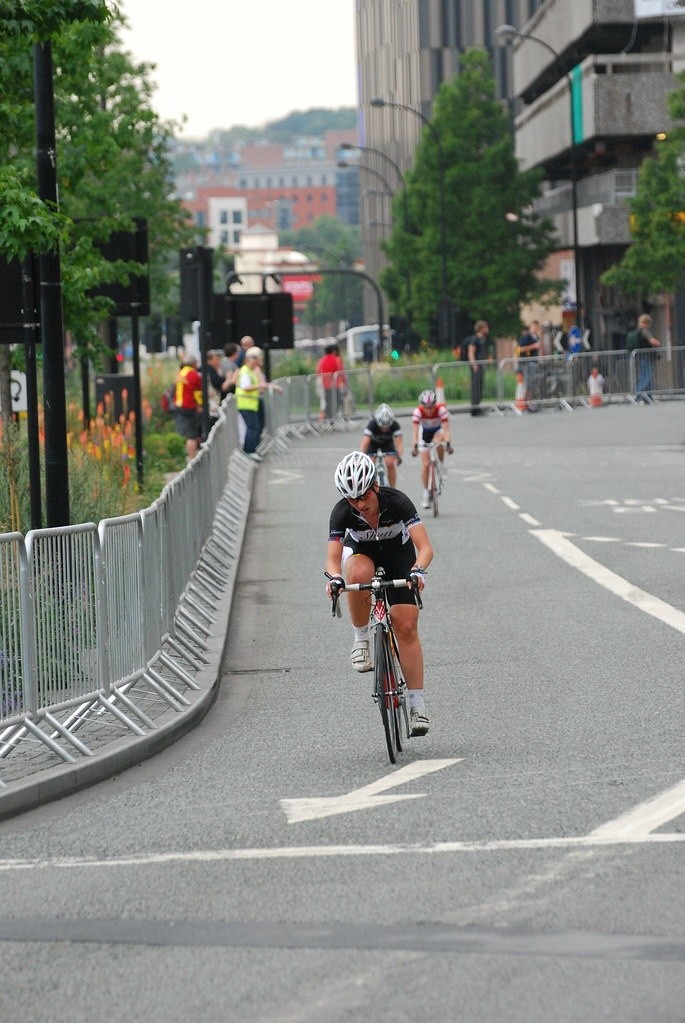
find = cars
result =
[296,324,390,370]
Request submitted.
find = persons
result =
[325,451,434,732]
[314,345,360,432]
[411,389,454,508]
[465,320,494,417]
[161,336,254,460]
[629,313,661,406]
[513,320,609,398]
[232,346,285,464]
[359,403,404,489]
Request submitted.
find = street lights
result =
[369,99,449,350]
[340,142,411,326]
[336,161,395,198]
[495,25,581,389]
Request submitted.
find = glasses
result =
[349,490,372,504]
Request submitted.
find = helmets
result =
[417,389,436,407]
[334,450,375,499]
[374,403,395,428]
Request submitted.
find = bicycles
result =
[331,566,427,764]
[367,449,402,487]
[412,442,454,518]
[525,360,562,412]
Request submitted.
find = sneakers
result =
[409,707,429,733]
[349,640,371,672]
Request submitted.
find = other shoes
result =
[439,465,448,480]
[248,453,263,462]
[421,492,432,508]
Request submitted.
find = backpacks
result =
[626,327,643,351]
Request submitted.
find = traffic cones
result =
[513,372,528,410]
[588,367,606,407]
